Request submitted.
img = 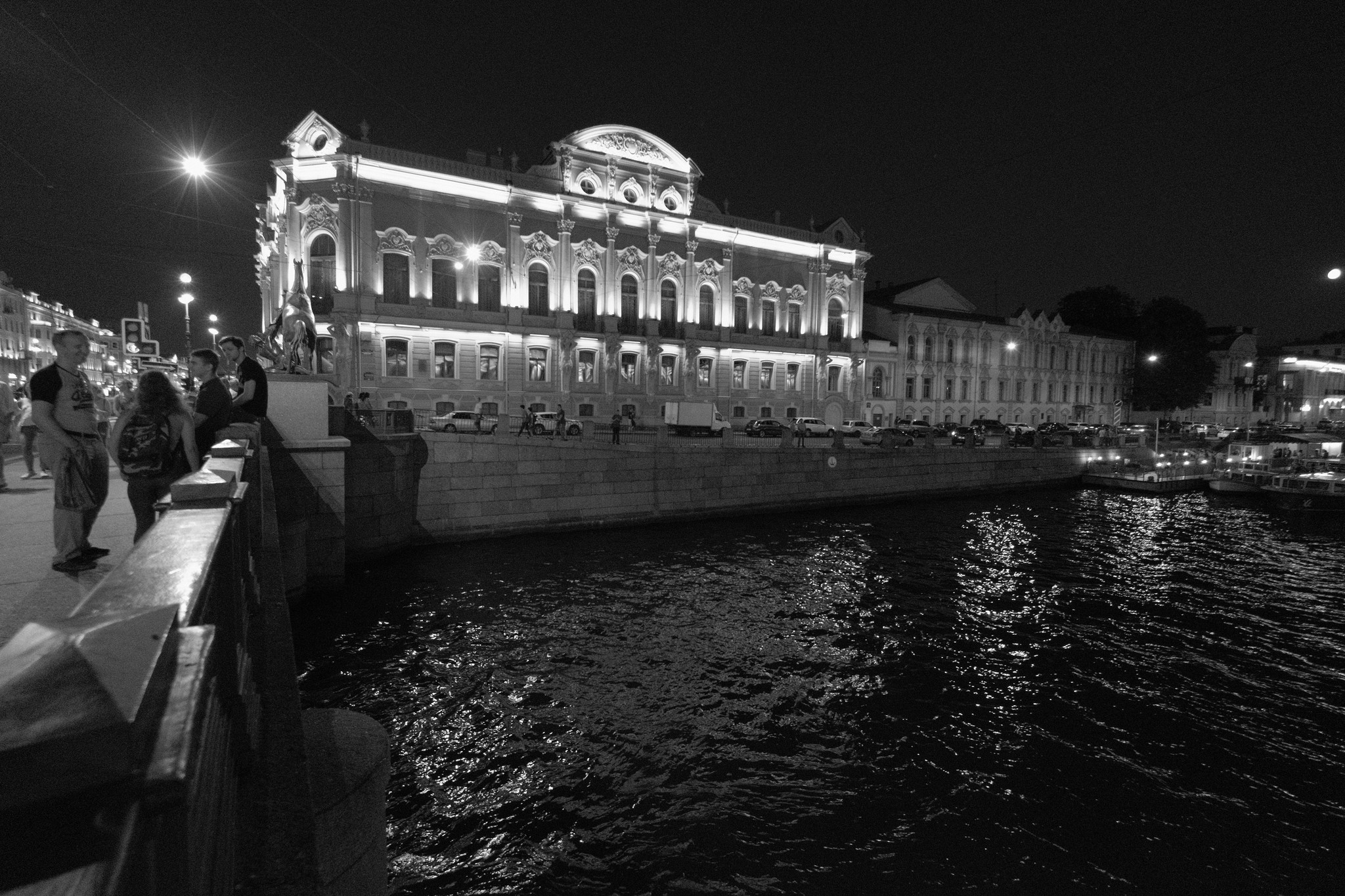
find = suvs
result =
[532,412,584,436]
[969,418,1014,440]
[788,417,836,438]
[897,419,938,438]
[745,419,791,437]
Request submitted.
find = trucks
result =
[664,400,732,437]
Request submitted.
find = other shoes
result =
[84,547,111,560]
[40,471,48,478]
[21,471,37,479]
[53,558,98,572]
[0,483,7,490]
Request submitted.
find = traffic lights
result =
[122,318,161,357]
[181,378,191,391]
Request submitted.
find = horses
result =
[267,257,318,376]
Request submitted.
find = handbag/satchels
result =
[611,423,616,431]
[106,422,112,438]
[53,446,100,511]
[355,403,359,409]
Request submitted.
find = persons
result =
[1004,428,1011,444]
[344,392,354,412]
[1098,428,1105,446]
[611,410,623,444]
[17,382,49,479]
[894,416,901,429]
[355,392,375,426]
[1014,425,1022,448]
[113,380,135,417]
[517,405,534,438]
[1105,429,1115,448]
[245,321,313,376]
[548,404,566,441]
[0,381,22,489]
[188,349,233,468]
[474,397,483,434]
[1272,446,1329,459]
[218,336,268,424]
[220,378,239,399]
[797,419,807,447]
[628,409,635,431]
[30,331,111,572]
[791,418,797,444]
[109,372,199,546]
[91,384,111,448]
[980,425,988,446]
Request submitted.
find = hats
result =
[347,392,354,397]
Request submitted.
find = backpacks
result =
[116,407,181,476]
[529,412,538,422]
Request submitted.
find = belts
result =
[64,428,101,440]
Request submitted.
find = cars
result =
[427,411,498,435]
[950,426,986,445]
[1029,418,1345,445]
[1005,422,1037,436]
[860,427,914,446]
[933,422,964,435]
[841,420,877,437]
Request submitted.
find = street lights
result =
[178,272,195,393]
[208,314,219,350]
